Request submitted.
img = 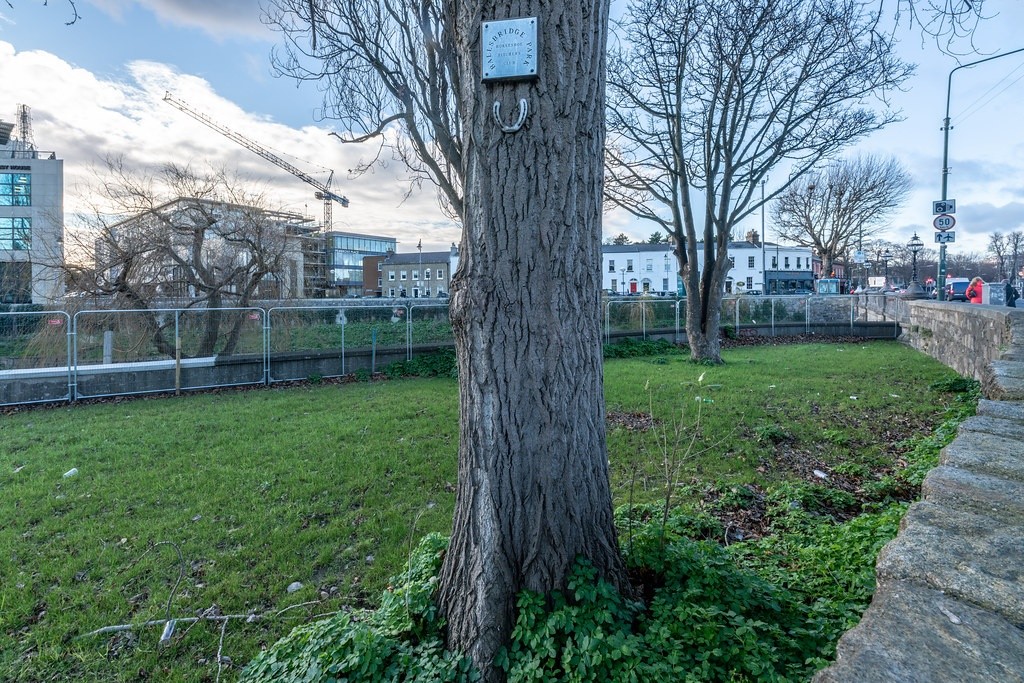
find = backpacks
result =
[1011,287,1020,299]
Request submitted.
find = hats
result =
[1001,279,1010,285]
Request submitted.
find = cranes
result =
[162,90,350,302]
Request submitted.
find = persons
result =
[1001,279,1021,308]
[850,285,854,294]
[966,277,986,304]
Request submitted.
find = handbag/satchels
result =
[968,285,976,298]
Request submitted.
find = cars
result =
[744,290,762,295]
[945,281,971,302]
[794,288,817,294]
[931,287,948,299]
[602,288,624,296]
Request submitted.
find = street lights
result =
[859,203,897,251]
[877,247,896,294]
[901,230,930,300]
[858,258,874,295]
